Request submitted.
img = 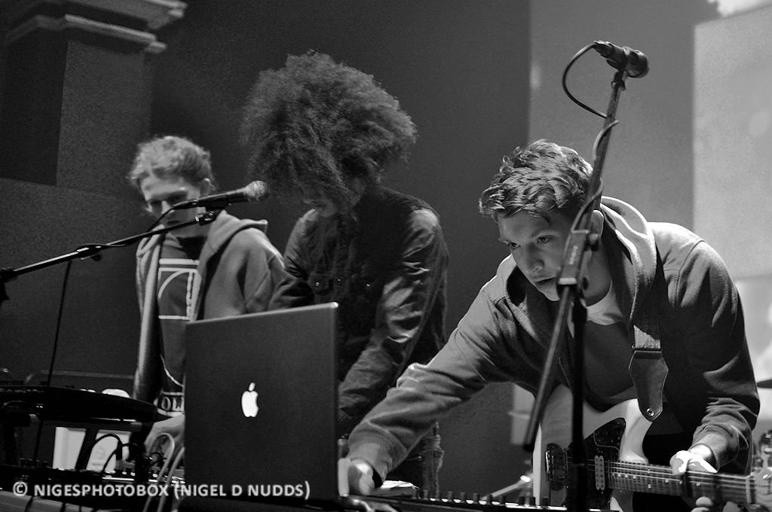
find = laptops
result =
[184,301,394,512]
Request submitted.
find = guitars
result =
[530,386,771,511]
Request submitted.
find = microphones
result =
[593,41,650,77]
[172,179,269,210]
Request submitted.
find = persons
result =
[337,140,760,512]
[127,135,286,481]
[243,48,450,496]
